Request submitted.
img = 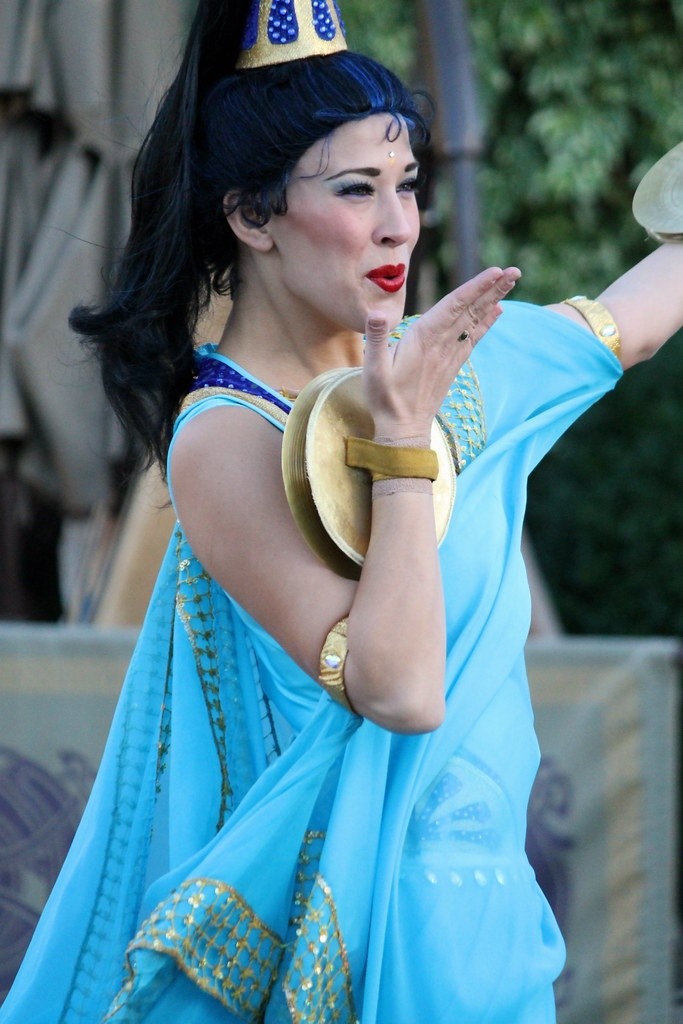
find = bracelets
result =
[370,436,435,499]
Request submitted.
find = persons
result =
[0,0,683,1024]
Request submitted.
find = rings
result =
[458,330,470,341]
